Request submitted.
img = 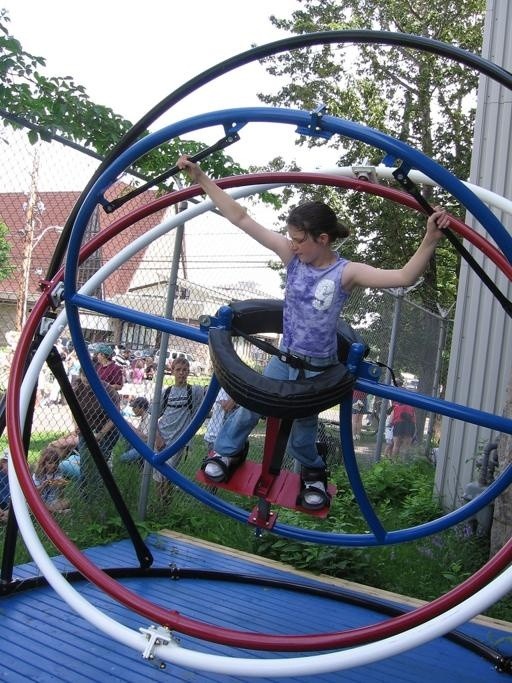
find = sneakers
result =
[205,452,232,477]
[304,479,325,504]
[352,435,360,440]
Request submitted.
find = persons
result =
[383,415,394,458]
[176,151,451,508]
[388,399,415,462]
[351,388,369,440]
[2,329,242,524]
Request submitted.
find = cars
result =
[402,379,419,392]
[54,337,206,379]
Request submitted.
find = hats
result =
[97,343,116,357]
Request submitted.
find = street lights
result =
[12,224,67,366]
